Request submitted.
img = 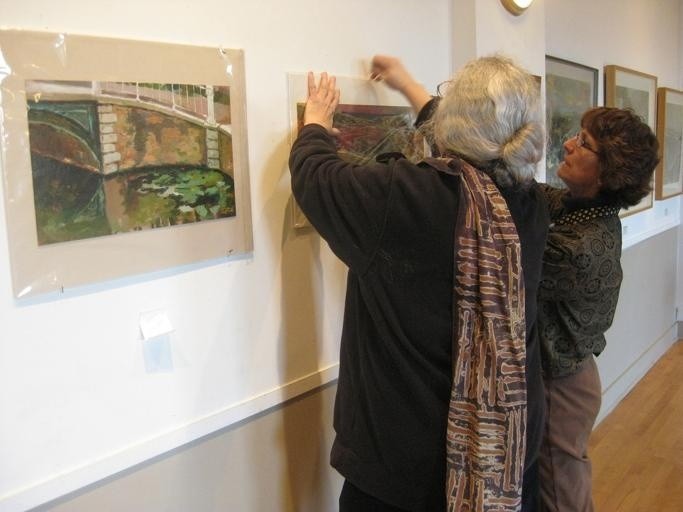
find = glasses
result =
[437,81,449,98]
[576,133,599,155]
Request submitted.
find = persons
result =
[285,50,552,512]
[528,106,662,510]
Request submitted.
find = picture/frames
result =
[545,54,683,218]
